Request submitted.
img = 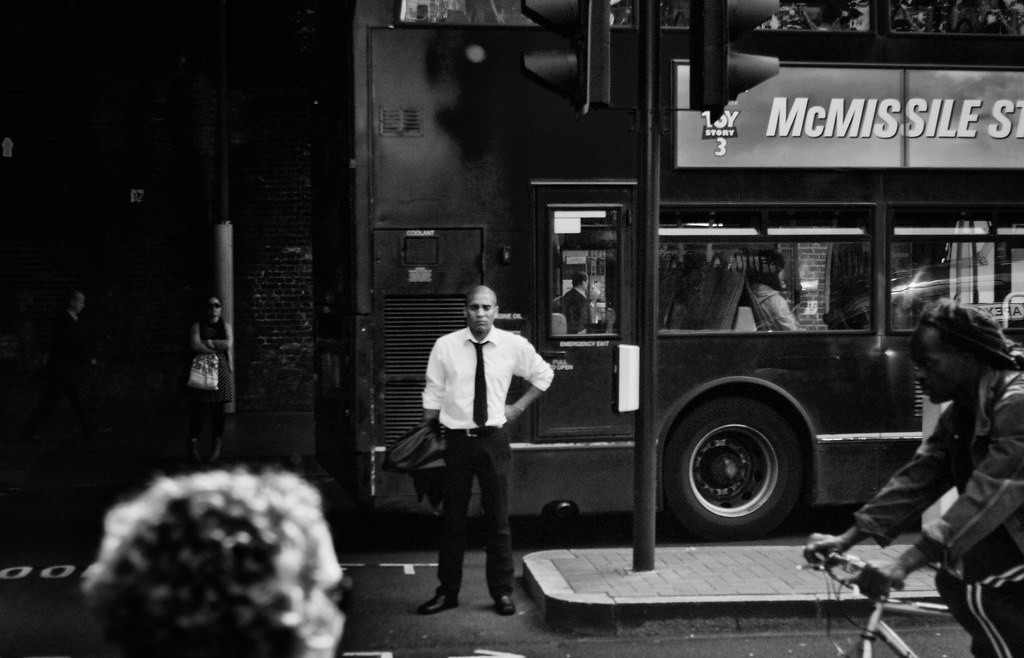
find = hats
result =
[918,298,1019,368]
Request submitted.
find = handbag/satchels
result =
[187,351,220,391]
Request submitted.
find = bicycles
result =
[806,527,934,656]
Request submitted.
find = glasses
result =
[207,302,222,309]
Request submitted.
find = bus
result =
[311,6,1017,536]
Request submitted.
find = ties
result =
[469,339,489,427]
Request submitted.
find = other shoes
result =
[207,438,222,462]
[187,438,205,466]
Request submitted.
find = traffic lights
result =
[528,1,785,121]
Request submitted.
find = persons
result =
[418,285,555,615]
[187,296,232,460]
[801,296,1024,658]
[563,269,589,335]
[22,288,114,442]
[754,249,806,332]
[80,469,348,656]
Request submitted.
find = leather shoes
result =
[416,594,458,614]
[495,595,516,615]
